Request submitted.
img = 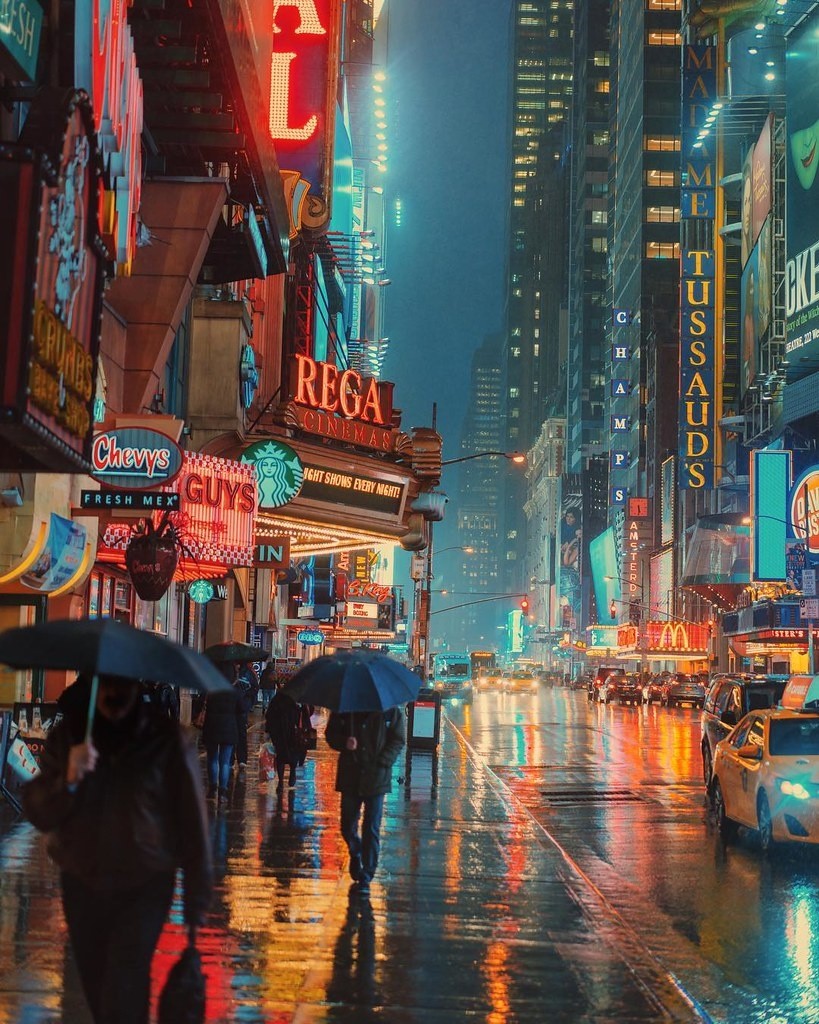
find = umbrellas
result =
[201,637,270,665]
[277,648,424,756]
[0,615,236,791]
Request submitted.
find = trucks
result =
[433,654,473,704]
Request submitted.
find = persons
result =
[325,704,406,883]
[194,663,319,805]
[19,679,216,1024]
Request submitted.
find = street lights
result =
[425,451,526,684]
[423,546,474,681]
[427,590,448,680]
[741,483,810,570]
[603,559,644,620]
[427,402,525,682]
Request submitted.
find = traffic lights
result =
[521,600,528,616]
[610,605,617,619]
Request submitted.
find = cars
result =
[701,676,788,788]
[477,663,555,694]
[705,672,760,704]
[569,675,593,691]
[710,710,819,851]
[641,671,672,706]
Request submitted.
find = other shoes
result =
[277,770,284,781]
[299,758,305,766]
[350,853,362,880]
[358,866,374,885]
[217,786,228,803]
[205,784,217,801]
[238,761,246,769]
[289,773,296,786]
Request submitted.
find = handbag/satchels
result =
[301,716,317,749]
[288,710,305,753]
[156,932,205,1024]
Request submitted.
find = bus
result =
[468,651,496,687]
[428,652,445,680]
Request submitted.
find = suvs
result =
[598,673,642,706]
[588,668,626,701]
[659,672,704,709]
